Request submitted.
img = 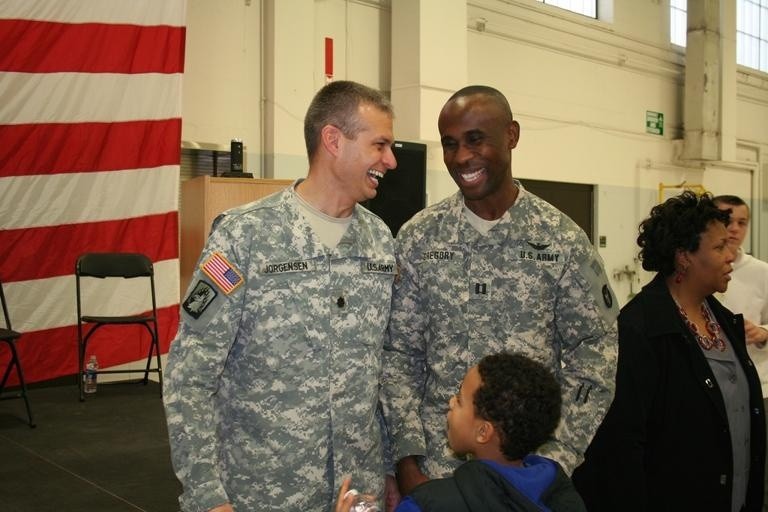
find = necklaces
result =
[677,302,725,351]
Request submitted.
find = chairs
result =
[0,280,35,428]
[76,253,163,401]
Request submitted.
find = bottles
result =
[343,489,382,512]
[230,138,244,172]
[84,355,100,393]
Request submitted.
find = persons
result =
[332,352,591,512]
[376,86,623,501]
[159,79,402,511]
[706,193,768,418]
[572,188,767,512]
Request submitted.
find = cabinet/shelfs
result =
[181,175,297,308]
